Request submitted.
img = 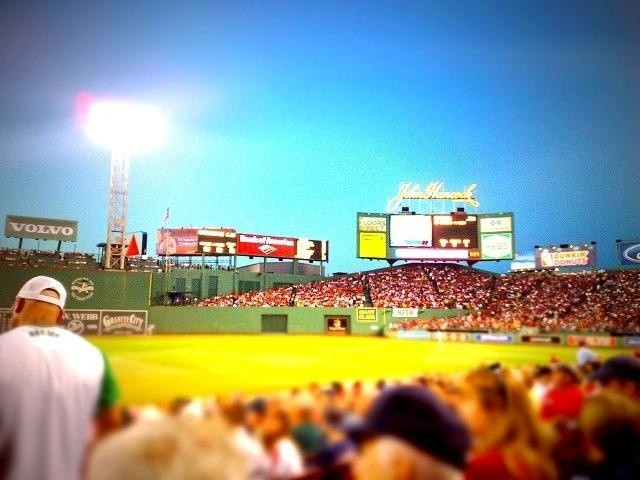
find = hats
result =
[81,414,255,480]
[346,384,471,472]
[586,354,640,385]
[15,275,67,312]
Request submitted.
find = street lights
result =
[86,102,169,271]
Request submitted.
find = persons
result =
[393,266,640,335]
[86,340,639,478]
[2,276,123,479]
[1,248,96,268]
[157,260,493,309]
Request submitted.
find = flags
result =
[165,208,169,224]
